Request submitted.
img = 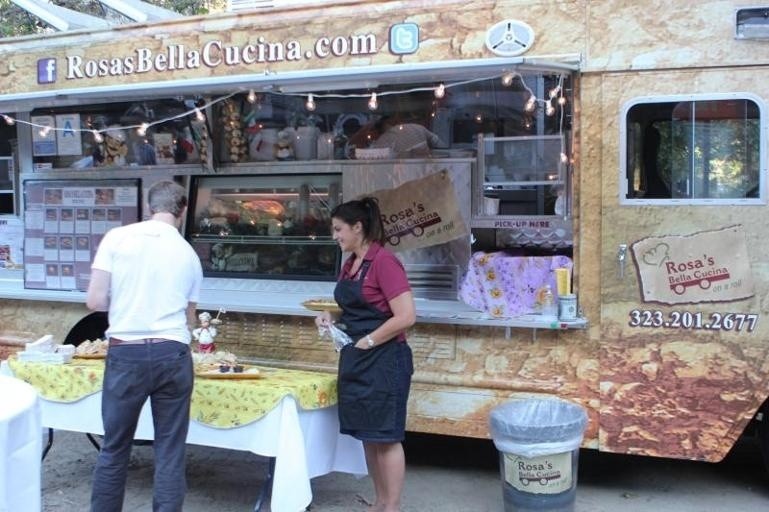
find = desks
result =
[2,346,370,512]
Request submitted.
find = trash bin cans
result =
[490,398,587,512]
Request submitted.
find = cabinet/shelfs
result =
[477,129,570,218]
[37,148,480,282]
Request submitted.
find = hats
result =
[106,124,127,142]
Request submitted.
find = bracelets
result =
[366,334,377,350]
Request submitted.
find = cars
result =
[118,100,195,130]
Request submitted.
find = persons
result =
[86,175,204,512]
[315,192,417,512]
[192,311,219,355]
[370,112,446,156]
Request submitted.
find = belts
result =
[109,337,167,347]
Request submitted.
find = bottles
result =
[541,284,578,321]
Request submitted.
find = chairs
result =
[38,308,108,460]
